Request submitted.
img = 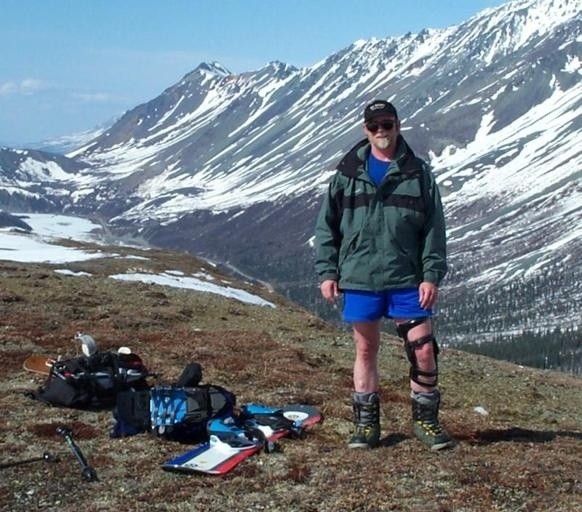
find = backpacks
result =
[141,381,236,444]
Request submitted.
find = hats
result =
[363,99,397,123]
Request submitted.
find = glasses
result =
[366,119,397,132]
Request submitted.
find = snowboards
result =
[160,405,324,475]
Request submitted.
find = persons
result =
[315,99,454,451]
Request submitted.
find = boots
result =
[410,388,456,452]
[345,390,381,450]
